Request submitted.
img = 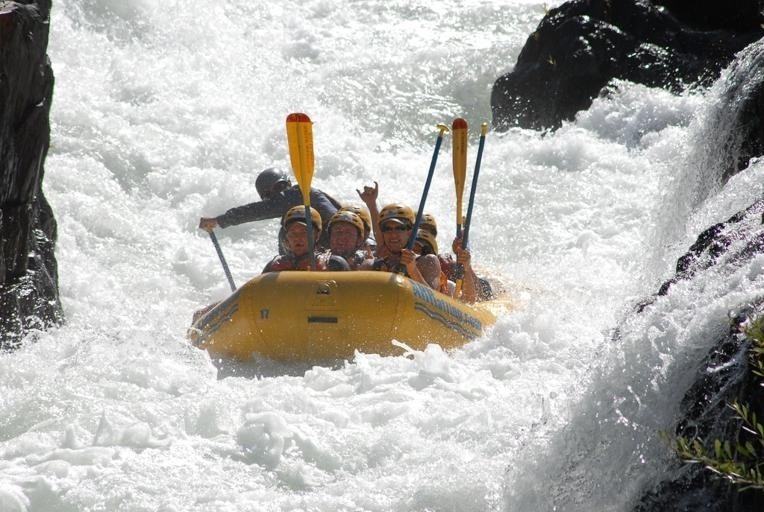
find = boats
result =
[186,271,495,374]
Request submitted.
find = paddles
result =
[453,122,488,299]
[286,113,314,270]
[452,119,467,262]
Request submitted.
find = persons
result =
[257,202,349,275]
[412,229,478,307]
[355,180,442,291]
[197,166,344,248]
[417,214,465,255]
[325,209,364,271]
[339,202,374,262]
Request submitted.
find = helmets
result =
[255,168,293,197]
[377,202,438,255]
[328,204,371,240]
[284,205,322,230]
